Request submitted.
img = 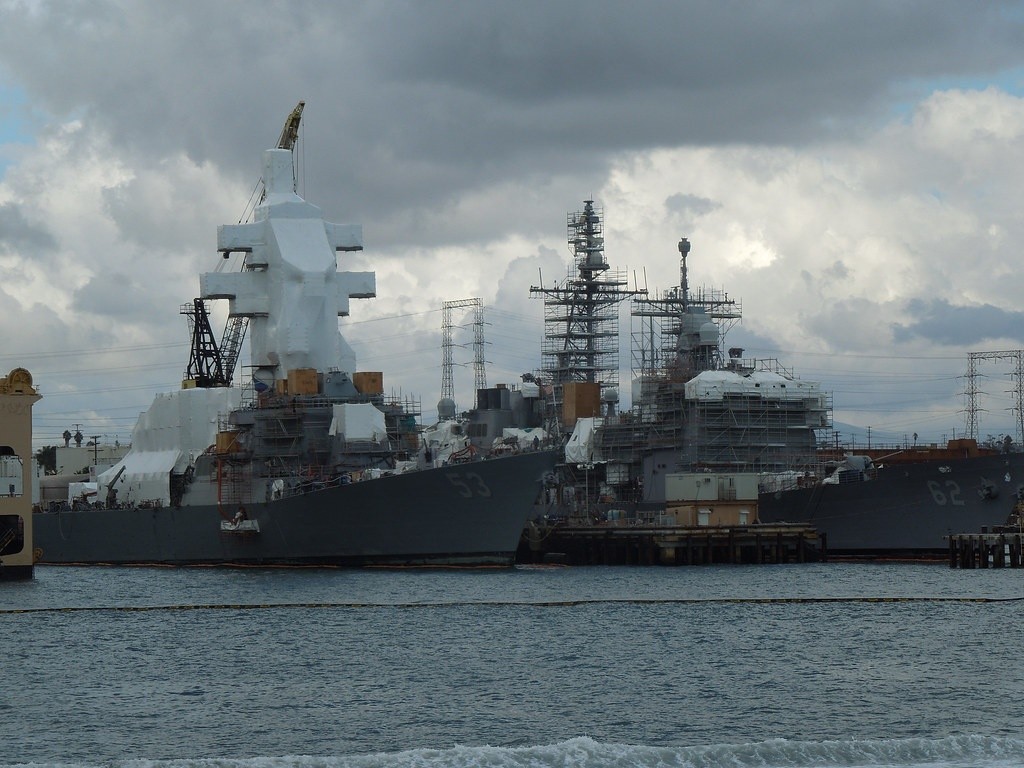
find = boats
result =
[419,192,1024,565]
[32,148,565,571]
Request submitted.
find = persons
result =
[533,435,540,450]
